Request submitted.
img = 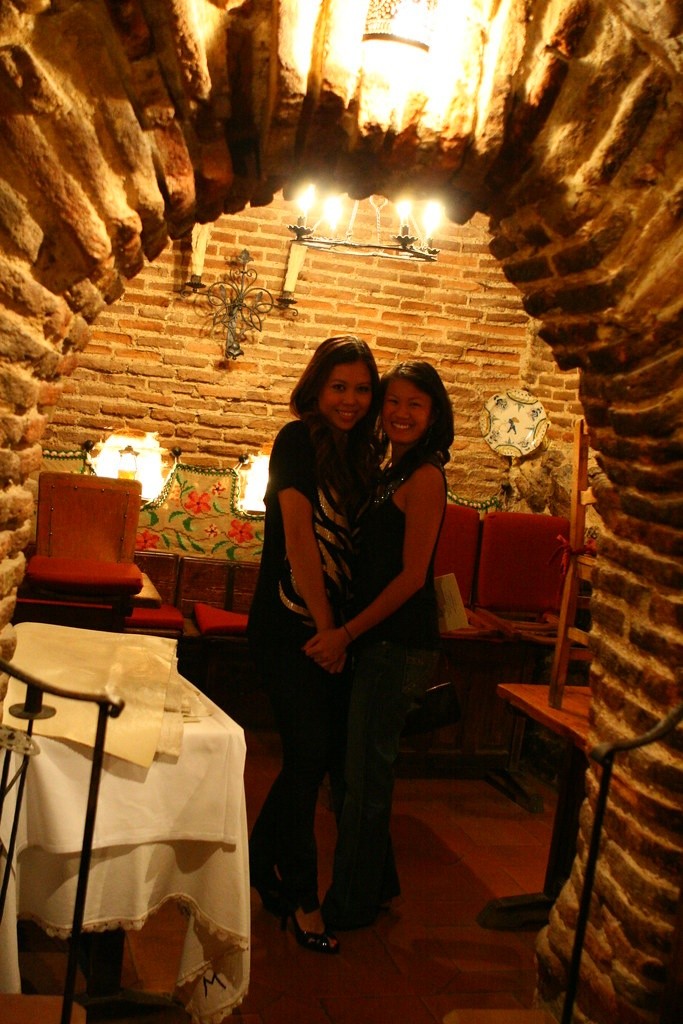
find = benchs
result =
[22,551,258,693]
[431,504,591,808]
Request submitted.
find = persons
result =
[249,340,455,954]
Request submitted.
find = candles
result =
[283,243,309,294]
[188,222,214,276]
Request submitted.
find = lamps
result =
[363,1,436,58]
[287,189,443,263]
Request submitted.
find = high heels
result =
[249,841,282,918]
[280,893,340,954]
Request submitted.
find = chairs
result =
[24,468,145,621]
[546,418,595,710]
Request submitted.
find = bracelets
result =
[343,624,354,641]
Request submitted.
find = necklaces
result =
[337,433,348,456]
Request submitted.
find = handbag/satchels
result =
[388,647,464,739]
[431,572,469,633]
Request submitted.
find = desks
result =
[477,679,597,933]
[0,627,253,1024]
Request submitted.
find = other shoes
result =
[314,871,351,930]
[350,897,391,928]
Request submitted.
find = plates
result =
[479,389,548,457]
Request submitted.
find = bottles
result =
[119,446,140,480]
